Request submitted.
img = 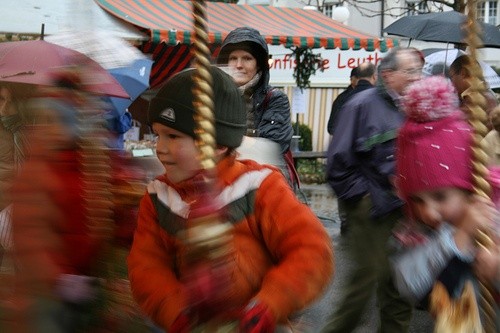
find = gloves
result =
[235,302,279,333]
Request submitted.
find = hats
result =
[146,65,245,147]
[26,68,86,135]
[393,74,476,200]
[224,41,266,74]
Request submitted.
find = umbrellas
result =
[386,10,500,81]
[421,48,500,89]
[0,38,129,99]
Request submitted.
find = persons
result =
[0,81,158,333]
[129,64,332,333]
[318,45,424,332]
[444,54,500,141]
[214,25,292,188]
[386,72,500,332]
[327,62,379,136]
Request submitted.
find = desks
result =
[290,150,329,193]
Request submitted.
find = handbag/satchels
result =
[262,87,300,192]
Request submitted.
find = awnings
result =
[97,0,401,84]
